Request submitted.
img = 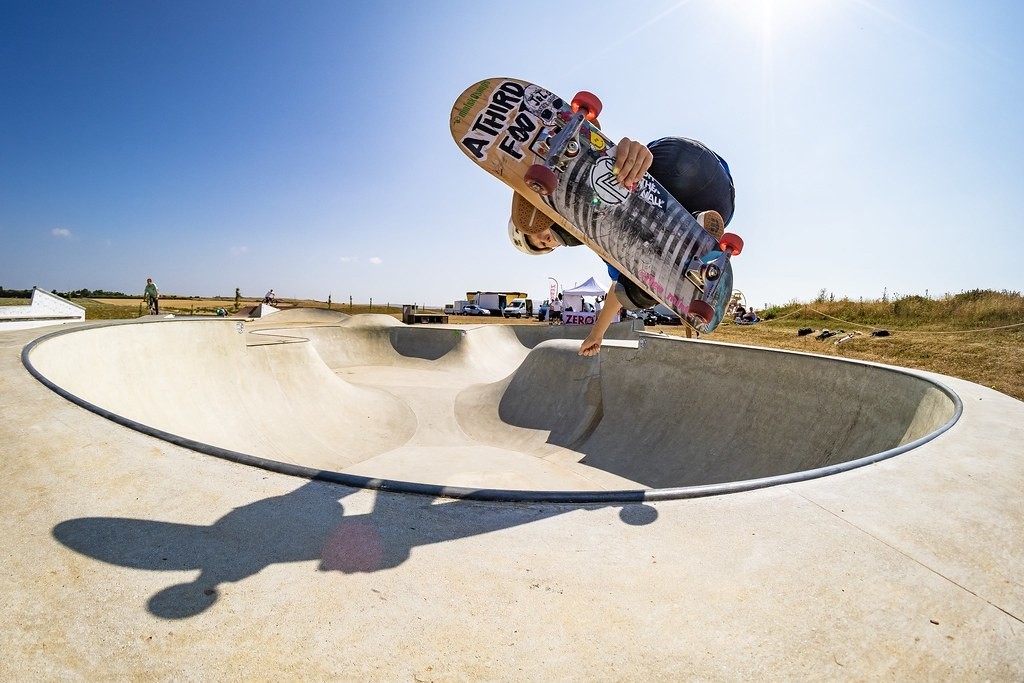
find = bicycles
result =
[142,298,156,315]
[262,294,278,307]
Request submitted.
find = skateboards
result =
[834,333,855,344]
[448,76,743,335]
[815,332,836,340]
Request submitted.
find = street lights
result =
[548,276,558,297]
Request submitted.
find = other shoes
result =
[692,210,724,241]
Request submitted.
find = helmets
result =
[508,216,553,255]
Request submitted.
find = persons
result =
[144,278,160,315]
[218,309,228,316]
[734,303,757,322]
[235,288,242,309]
[265,289,274,306]
[508,135,736,356]
[550,296,601,326]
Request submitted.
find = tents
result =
[563,276,620,324]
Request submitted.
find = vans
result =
[503,298,542,319]
[444,304,454,315]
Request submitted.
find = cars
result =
[460,304,491,316]
[539,300,551,321]
